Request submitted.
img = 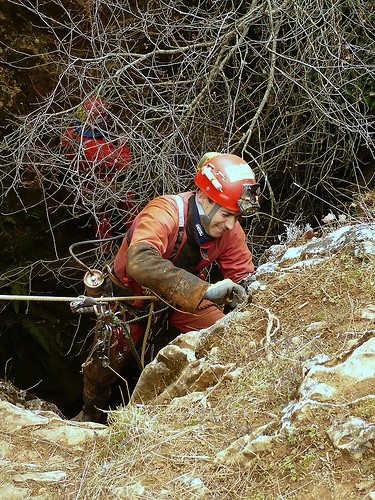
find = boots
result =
[64,379,108,426]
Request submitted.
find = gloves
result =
[202,276,246,312]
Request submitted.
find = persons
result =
[70,151,262,424]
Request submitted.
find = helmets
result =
[76,96,112,128]
[193,152,258,214]
